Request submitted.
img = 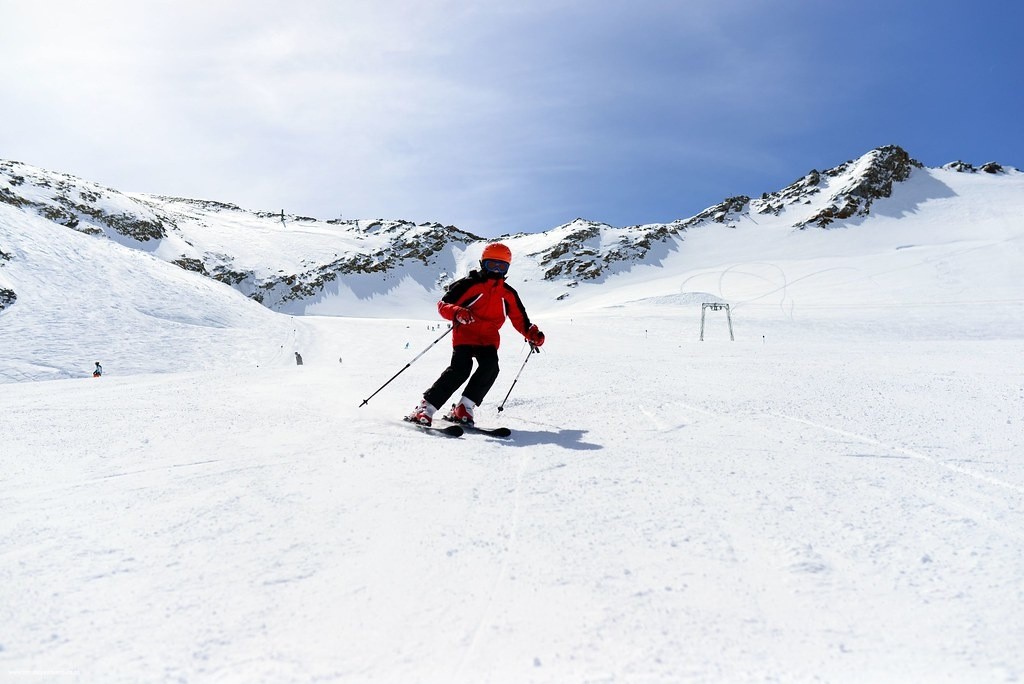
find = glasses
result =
[484,261,508,272]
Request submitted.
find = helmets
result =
[481,243,511,264]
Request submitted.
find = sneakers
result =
[451,403,474,425]
[410,400,433,427]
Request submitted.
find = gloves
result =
[456,308,476,324]
[527,324,545,346]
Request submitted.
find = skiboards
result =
[391,415,511,439]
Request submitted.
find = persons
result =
[93,362,103,378]
[411,243,544,429]
[295,351,302,365]
